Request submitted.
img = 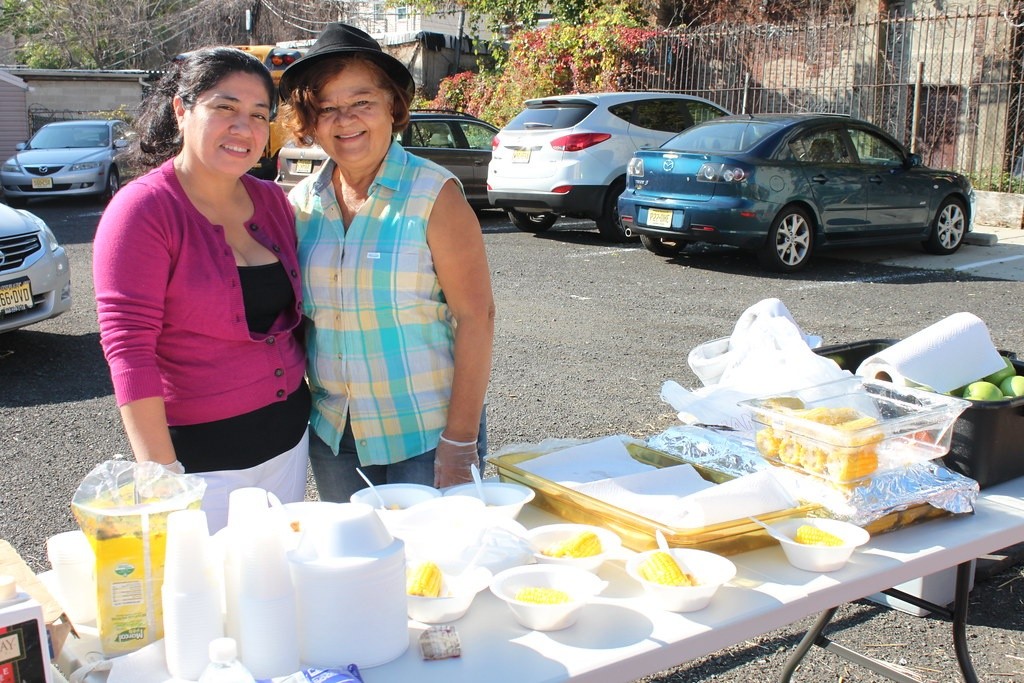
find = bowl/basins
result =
[737,376,973,484]
[292,504,409,667]
[625,549,737,611]
[405,568,492,623]
[490,563,609,631]
[528,524,622,570]
[443,483,535,519]
[768,517,871,572]
[350,483,442,512]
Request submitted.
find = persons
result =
[276,22,496,504]
[92,47,312,536]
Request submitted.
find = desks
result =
[34,446,1024,683]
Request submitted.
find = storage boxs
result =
[805,338,1023,488]
[743,379,973,486]
[864,561,978,620]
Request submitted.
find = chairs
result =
[429,130,448,148]
[804,139,835,162]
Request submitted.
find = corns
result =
[551,530,603,557]
[756,407,884,484]
[795,524,844,545]
[640,551,692,587]
[407,561,443,597]
[514,586,569,605]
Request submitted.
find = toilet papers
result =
[852,309,1010,396]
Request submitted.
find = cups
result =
[162,488,297,676]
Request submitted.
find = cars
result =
[617,112,976,274]
[0,202,73,331]
[486,91,736,244]
[0,120,141,207]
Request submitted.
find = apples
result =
[945,355,1024,401]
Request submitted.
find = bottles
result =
[198,637,255,683]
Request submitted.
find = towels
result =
[659,298,843,431]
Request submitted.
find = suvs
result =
[275,108,501,216]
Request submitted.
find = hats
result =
[277,22,416,109]
